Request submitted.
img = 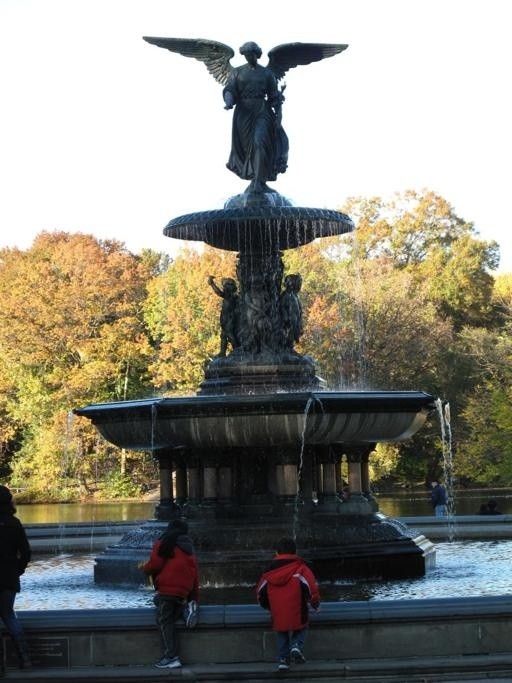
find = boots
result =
[14,631,31,668]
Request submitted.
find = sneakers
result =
[289,644,307,662]
[278,658,289,669]
[154,654,181,669]
[186,601,199,630]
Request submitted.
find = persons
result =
[429,480,446,516]
[478,501,501,513]
[223,43,289,194]
[208,274,305,357]
[258,537,321,670]
[0,485,31,669]
[137,521,201,670]
